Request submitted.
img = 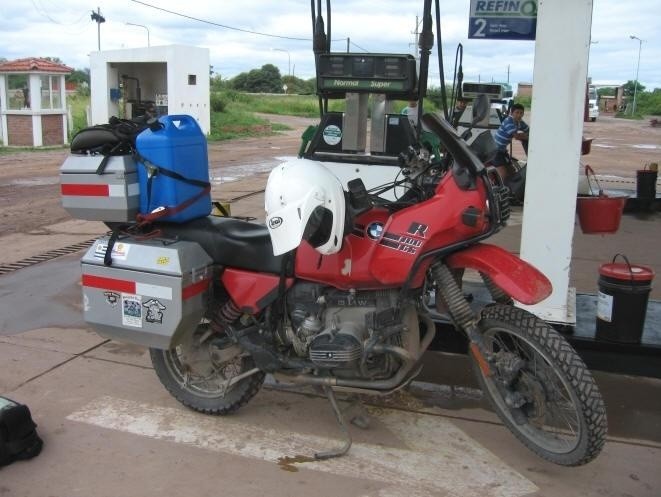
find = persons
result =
[401,100,424,120]
[494,104,529,182]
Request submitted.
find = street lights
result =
[125,22,150,49]
[630,34,648,118]
[272,47,291,76]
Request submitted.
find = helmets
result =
[264,159,346,256]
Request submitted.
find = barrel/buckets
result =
[576,165,629,234]
[136,115,211,224]
[637,163,657,201]
[596,252,654,345]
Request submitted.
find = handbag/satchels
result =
[70,122,143,153]
[1,404,43,467]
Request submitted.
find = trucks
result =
[588,84,599,122]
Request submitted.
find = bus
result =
[467,82,514,116]
